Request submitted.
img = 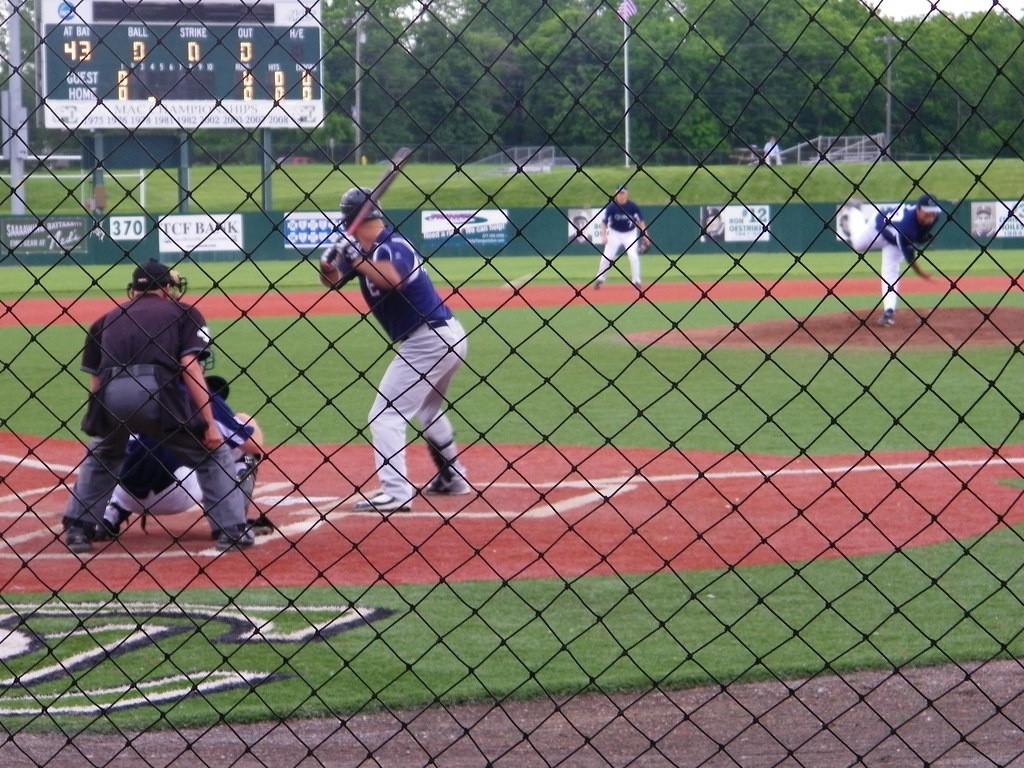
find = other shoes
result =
[594,282,601,289]
[878,312,893,327]
[635,283,641,289]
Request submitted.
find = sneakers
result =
[245,518,274,537]
[91,520,114,540]
[426,473,471,494]
[65,528,91,552]
[216,528,256,551]
[354,493,410,512]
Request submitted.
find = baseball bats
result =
[328,146,412,262]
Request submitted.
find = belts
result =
[110,365,155,378]
[401,320,448,340]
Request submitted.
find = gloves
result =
[321,247,342,270]
[335,233,362,260]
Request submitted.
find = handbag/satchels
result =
[155,365,208,432]
[81,387,105,438]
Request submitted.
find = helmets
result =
[340,187,382,221]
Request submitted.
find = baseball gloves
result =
[918,233,934,244]
[638,238,651,254]
[205,376,230,403]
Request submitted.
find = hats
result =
[132,260,170,291]
[976,206,991,214]
[706,208,720,218]
[616,185,628,195]
[919,192,940,213]
[572,212,587,223]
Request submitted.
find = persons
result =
[321,186,468,512]
[62,258,257,550]
[763,136,782,166]
[843,195,941,328]
[94,377,265,539]
[592,185,649,290]
